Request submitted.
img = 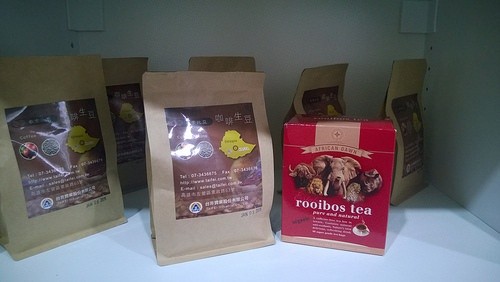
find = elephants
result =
[313,154,362,200]
